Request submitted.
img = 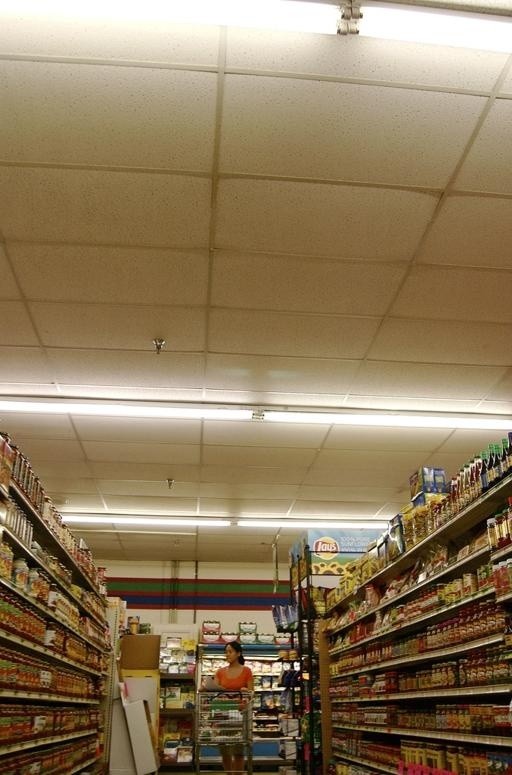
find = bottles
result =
[426,432,512,559]
[1,434,111,772]
[331,560,510,775]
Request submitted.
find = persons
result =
[212,640,253,775]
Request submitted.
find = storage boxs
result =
[408,464,449,495]
[410,492,441,505]
[196,614,299,647]
[120,630,164,673]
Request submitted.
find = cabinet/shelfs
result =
[0,472,123,774]
[156,633,202,774]
[110,669,158,775]
[196,637,299,767]
[309,469,512,775]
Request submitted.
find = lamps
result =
[0,391,512,438]
[61,505,394,534]
[2,0,507,52]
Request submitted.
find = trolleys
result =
[193,687,253,774]
